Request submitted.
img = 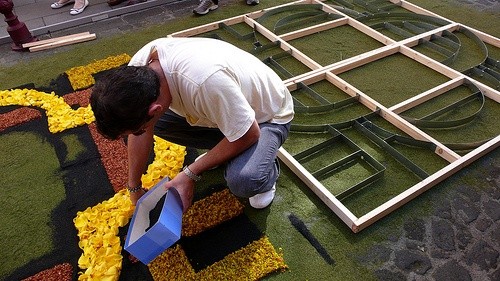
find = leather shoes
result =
[70,0,89,15]
[51,0,74,9]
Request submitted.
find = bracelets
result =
[127,181,142,193]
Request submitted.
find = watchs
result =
[183,165,202,183]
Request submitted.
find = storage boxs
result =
[123,177,183,267]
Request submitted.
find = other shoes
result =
[195,151,218,171]
[249,182,277,209]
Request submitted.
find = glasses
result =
[121,119,146,139]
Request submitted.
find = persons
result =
[192,0,260,14]
[106,0,148,7]
[51,0,89,15]
[90,37,295,208]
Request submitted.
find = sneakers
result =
[193,0,218,15]
[247,0,259,5]
